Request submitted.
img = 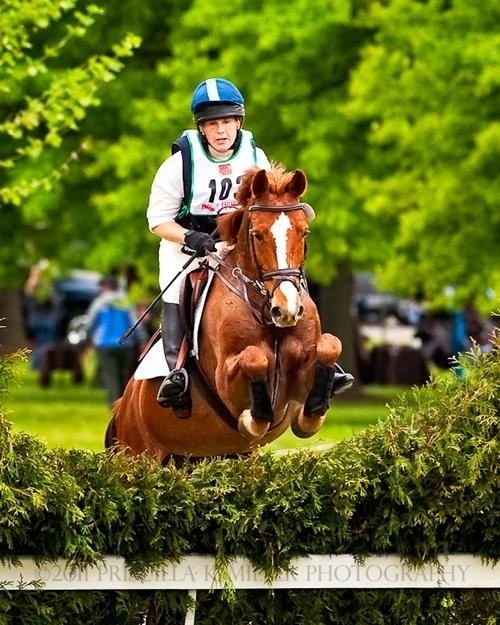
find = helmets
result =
[192,79,245,123]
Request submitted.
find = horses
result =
[103,159,345,472]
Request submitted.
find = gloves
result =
[185,231,217,252]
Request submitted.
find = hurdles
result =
[0,330,500,623]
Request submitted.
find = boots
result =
[330,373,355,399]
[160,302,192,418]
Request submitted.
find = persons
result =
[146,76,356,422]
[68,274,150,401]
[115,264,156,349]
